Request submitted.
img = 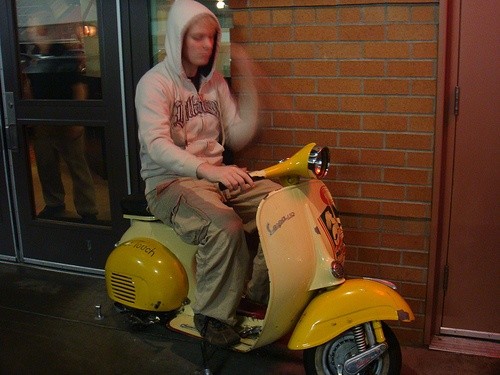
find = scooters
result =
[106,143,416,375]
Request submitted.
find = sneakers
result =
[192,312,241,347]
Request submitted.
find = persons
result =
[21,7,99,220]
[134,0,263,347]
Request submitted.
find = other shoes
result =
[38,204,61,216]
